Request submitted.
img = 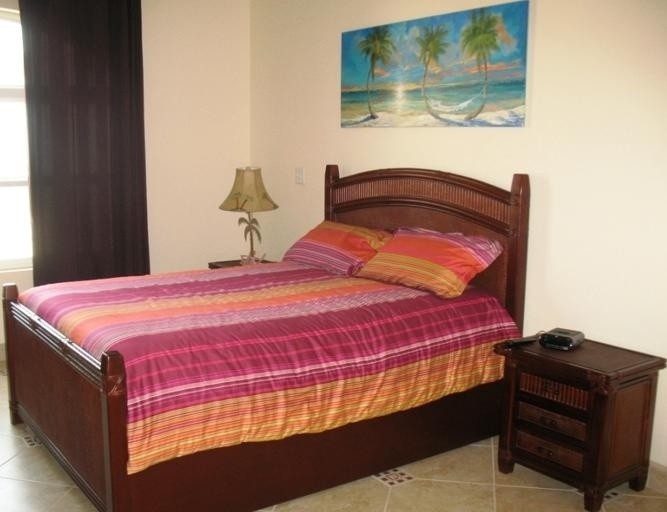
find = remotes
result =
[506,337,537,347]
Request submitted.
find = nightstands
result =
[208,260,278,270]
[494,333,667,512]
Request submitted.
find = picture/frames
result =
[340,0,531,130]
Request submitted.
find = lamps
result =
[218,168,278,265]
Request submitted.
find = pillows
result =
[281,220,389,276]
[355,226,503,298]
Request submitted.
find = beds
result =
[3,165,531,512]
